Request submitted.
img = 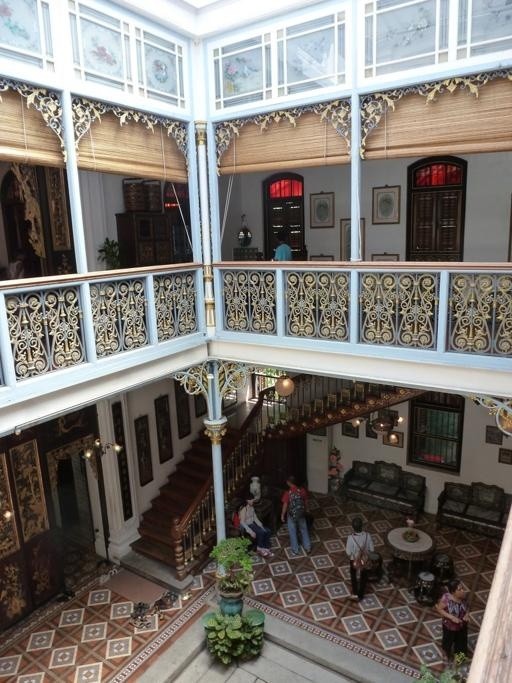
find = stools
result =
[365,551,383,582]
[414,570,438,606]
[430,552,454,585]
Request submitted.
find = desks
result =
[246,496,275,533]
[383,525,436,581]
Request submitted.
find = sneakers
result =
[306,548,312,553]
[294,550,299,554]
[350,594,358,600]
[264,549,275,556]
[257,547,268,556]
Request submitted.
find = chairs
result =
[225,502,262,551]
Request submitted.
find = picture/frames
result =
[133,373,237,486]
[370,253,398,261]
[371,184,400,224]
[339,409,404,449]
[309,191,335,227]
[485,425,503,444]
[340,217,365,261]
[310,255,333,260]
[498,447,511,464]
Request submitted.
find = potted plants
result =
[209,533,256,617]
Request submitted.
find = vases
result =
[250,475,263,502]
[331,477,342,491]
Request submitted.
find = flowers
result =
[329,443,345,478]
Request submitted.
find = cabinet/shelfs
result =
[172,223,193,262]
[115,209,173,267]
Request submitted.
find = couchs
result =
[436,479,511,537]
[334,460,427,521]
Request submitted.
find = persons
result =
[343,515,376,604]
[435,579,470,662]
[273,230,294,260]
[280,474,314,555]
[237,491,277,559]
[249,473,263,499]
[6,249,28,279]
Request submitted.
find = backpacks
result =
[349,531,372,571]
[232,504,247,529]
[287,487,306,520]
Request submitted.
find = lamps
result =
[273,372,296,396]
[355,385,404,443]
[78,434,125,460]
[235,228,253,252]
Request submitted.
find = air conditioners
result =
[305,421,332,495]
